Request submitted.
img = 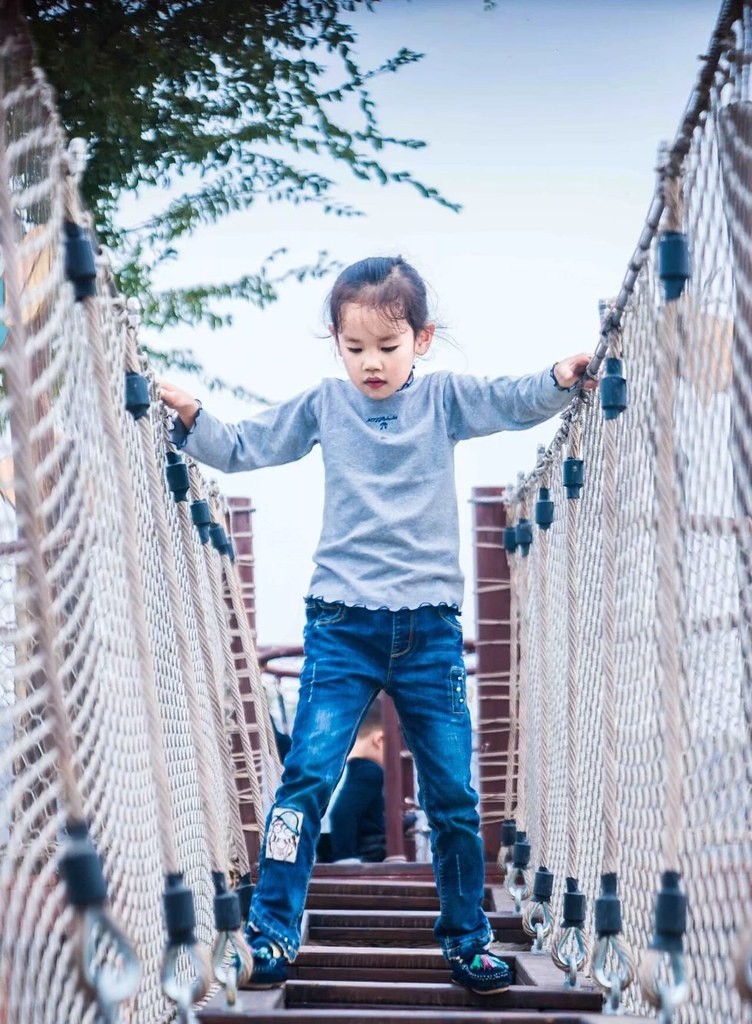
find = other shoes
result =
[228,941,290,991]
[448,949,511,995]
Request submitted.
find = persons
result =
[153,257,597,993]
[316,697,416,865]
